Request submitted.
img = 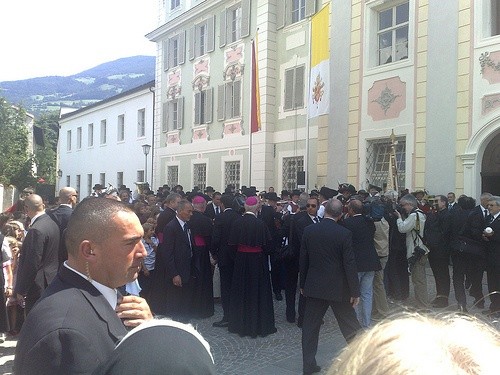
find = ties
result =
[216,206,219,214]
[313,218,319,223]
[484,210,487,218]
[117,289,131,331]
[448,203,452,211]
[490,215,494,222]
[183,223,188,238]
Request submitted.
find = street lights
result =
[141,143,152,182]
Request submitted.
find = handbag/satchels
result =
[275,214,296,264]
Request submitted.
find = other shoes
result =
[388,293,499,319]
[276,292,282,300]
[269,328,277,333]
[213,320,229,327]
[304,366,321,375]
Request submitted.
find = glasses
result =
[306,204,318,208]
[70,194,78,198]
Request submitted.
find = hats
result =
[92,184,129,190]
[157,183,386,218]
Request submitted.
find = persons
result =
[326,301,500,375]
[161,201,196,326]
[224,195,281,339]
[12,196,160,375]
[0,178,500,341]
[298,197,370,375]
[105,316,218,375]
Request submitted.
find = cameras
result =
[391,202,403,214]
[407,246,426,265]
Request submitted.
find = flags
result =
[310,3,329,118]
[251,33,261,134]
